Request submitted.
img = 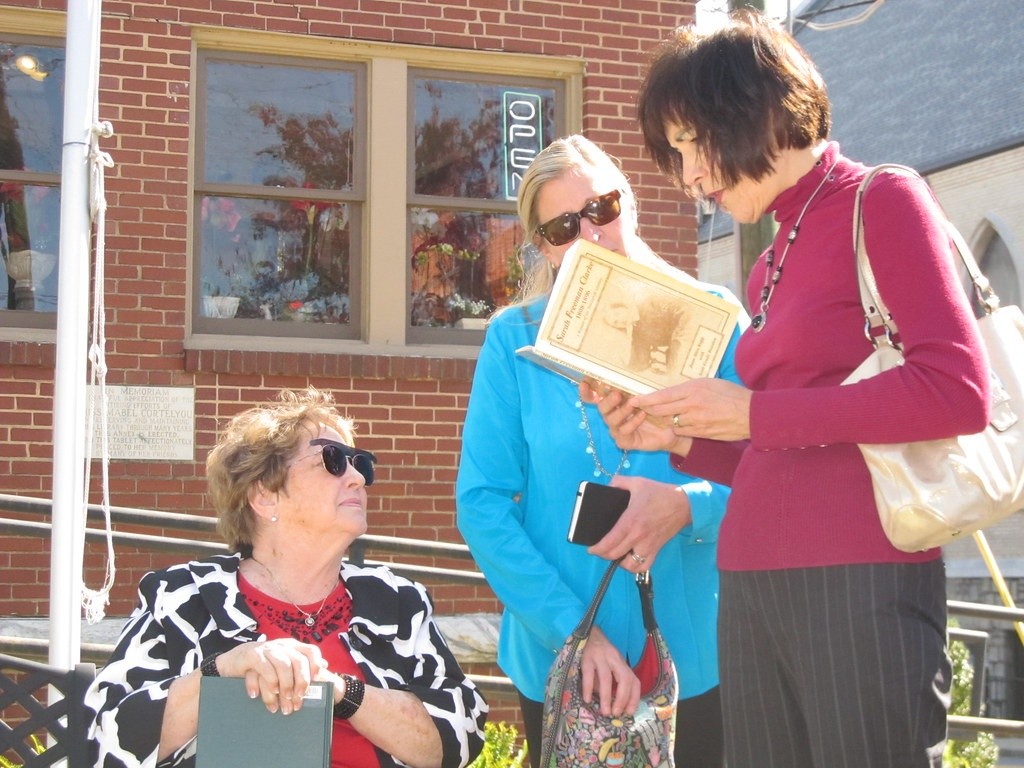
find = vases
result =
[4,250,57,292]
[203,297,242,319]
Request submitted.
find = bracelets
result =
[329,671,364,720]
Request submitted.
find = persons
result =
[83,387,488,768]
[456,134,750,768]
[634,4,991,768]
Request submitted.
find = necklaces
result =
[251,556,339,627]
[751,154,849,334]
[578,393,629,479]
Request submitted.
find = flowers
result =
[0,168,49,248]
[274,181,487,326]
[197,192,239,292]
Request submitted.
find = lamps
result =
[13,47,60,81]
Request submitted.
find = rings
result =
[673,414,679,428]
[630,549,646,566]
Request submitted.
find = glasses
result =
[285,444,375,487]
[535,189,621,247]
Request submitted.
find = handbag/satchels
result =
[539,554,680,768]
[840,162,1024,553]
